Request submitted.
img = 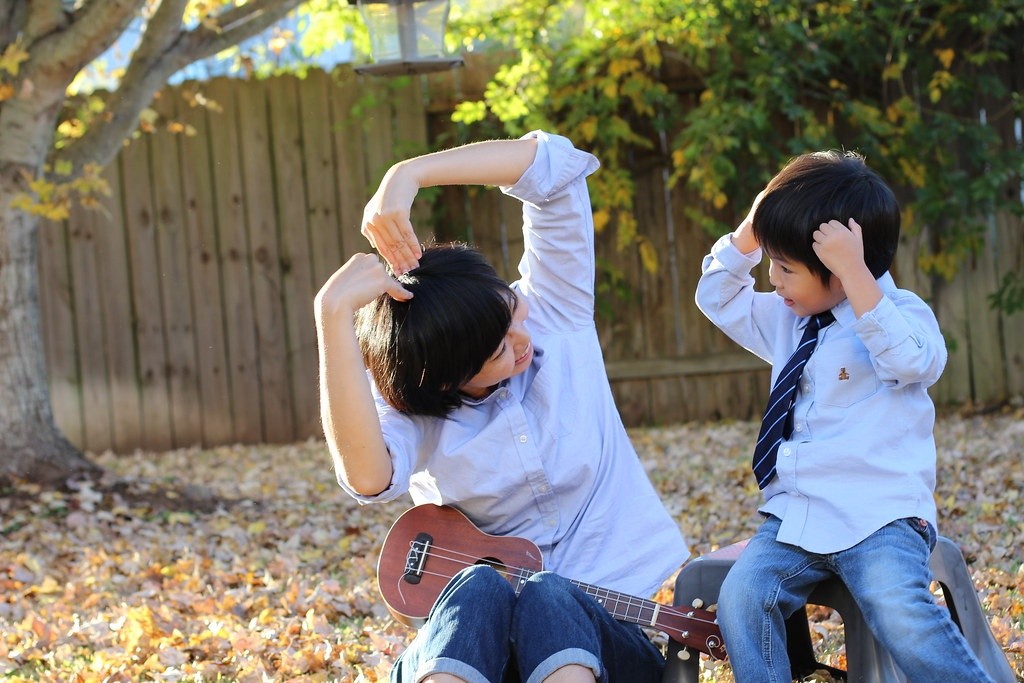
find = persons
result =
[314,128,692,682]
[695,148,995,683]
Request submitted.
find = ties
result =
[752,309,837,491]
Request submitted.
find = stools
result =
[664,536,1016,683]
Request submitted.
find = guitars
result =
[378,503,737,664]
